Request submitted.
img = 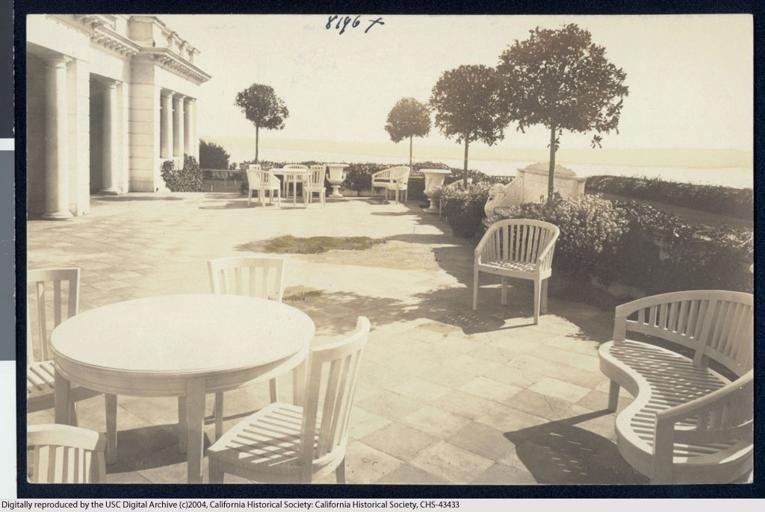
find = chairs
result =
[471,216,561,323]
[24,252,373,488]
[245,163,329,208]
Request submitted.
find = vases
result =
[326,163,350,198]
[419,168,451,204]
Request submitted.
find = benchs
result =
[598,289,757,487]
[371,165,410,205]
